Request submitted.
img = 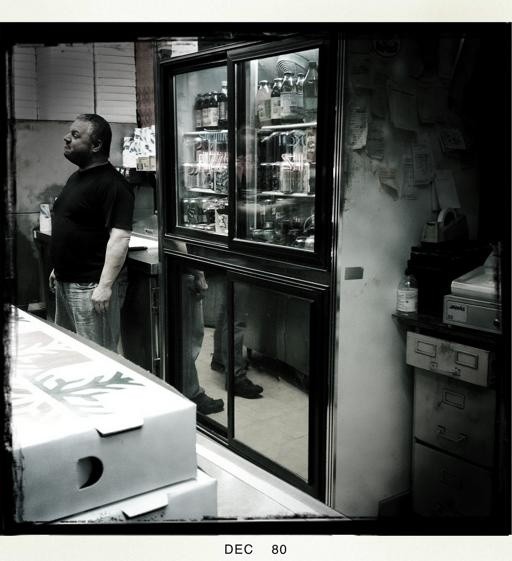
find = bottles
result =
[254,60,318,124]
[194,79,229,130]
[397,267,418,320]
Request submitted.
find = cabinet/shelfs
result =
[154,24,346,270]
[405,331,500,518]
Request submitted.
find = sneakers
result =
[191,392,224,413]
[211,359,226,372]
[225,377,263,396]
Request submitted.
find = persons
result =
[169,236,224,414]
[210,269,264,398]
[46,115,136,355]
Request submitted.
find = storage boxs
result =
[10,305,219,525]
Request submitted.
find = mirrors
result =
[157,248,330,506]
